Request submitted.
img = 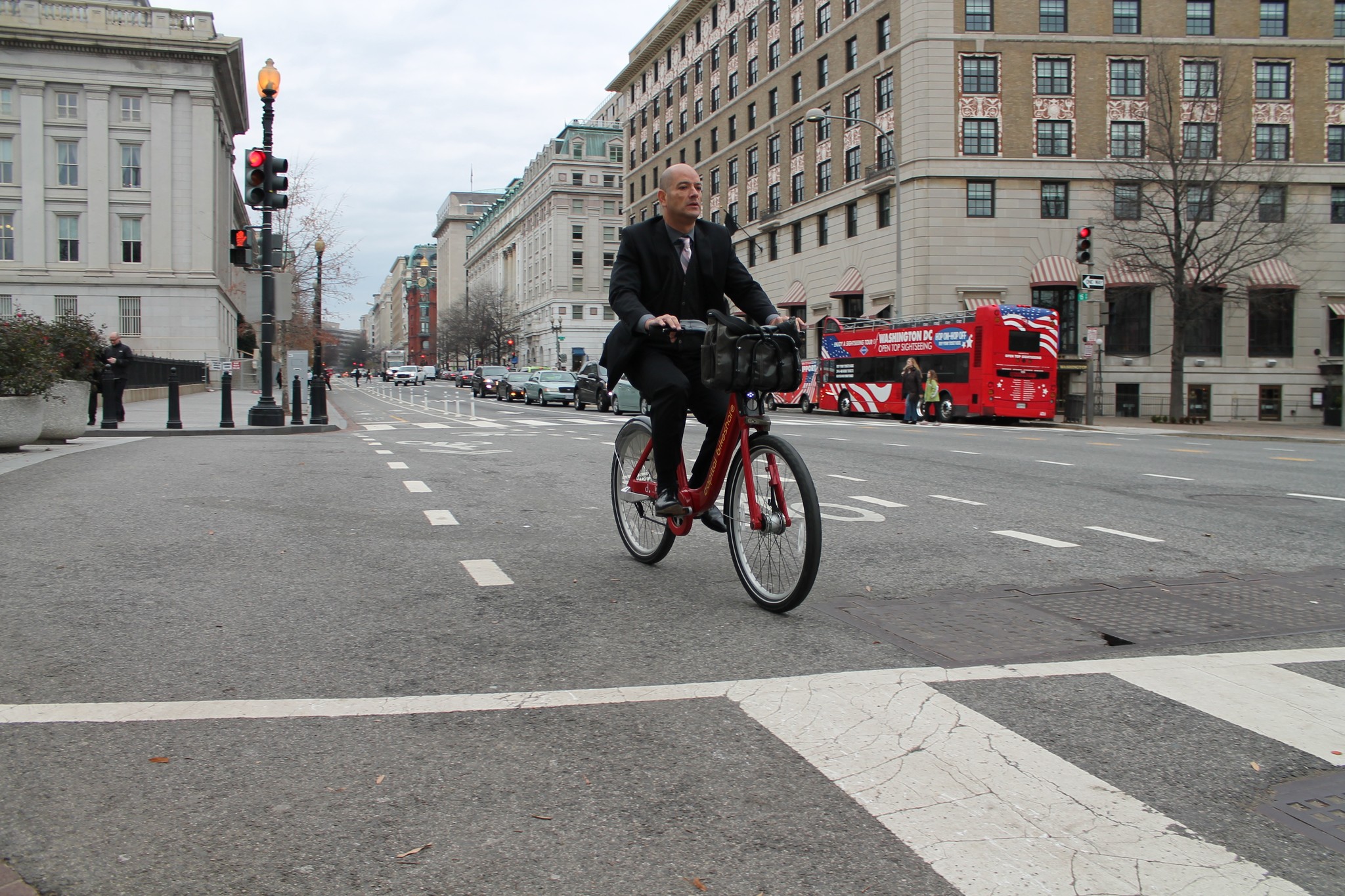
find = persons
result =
[276,368,282,390]
[323,370,332,391]
[87,331,125,426]
[355,367,361,387]
[920,369,943,426]
[598,164,806,535]
[365,369,372,383]
[100,332,134,422]
[899,358,923,425]
[308,370,313,388]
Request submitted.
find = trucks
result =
[380,350,406,382]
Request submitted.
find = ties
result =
[678,236,692,276]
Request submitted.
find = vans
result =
[422,365,435,382]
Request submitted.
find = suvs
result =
[471,364,509,400]
[573,358,613,413]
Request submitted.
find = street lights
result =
[248,59,287,422]
[550,315,562,372]
[310,236,330,427]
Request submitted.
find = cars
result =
[455,370,475,388]
[506,365,550,373]
[436,370,459,380]
[329,368,378,378]
[611,373,653,414]
[523,370,578,404]
[394,365,426,386]
[496,372,526,402]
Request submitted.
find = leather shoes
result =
[700,504,728,533]
[655,487,683,515]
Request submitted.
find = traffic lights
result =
[267,150,289,209]
[256,231,285,270]
[225,227,252,268]
[244,149,268,206]
[1076,224,1092,265]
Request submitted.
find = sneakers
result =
[932,421,941,426]
[919,419,929,425]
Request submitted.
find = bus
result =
[763,357,820,412]
[820,303,1058,423]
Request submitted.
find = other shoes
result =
[117,416,124,422]
[908,421,916,424]
[87,418,96,425]
[899,420,908,424]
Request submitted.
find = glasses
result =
[109,337,118,341]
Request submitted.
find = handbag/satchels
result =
[700,309,804,392]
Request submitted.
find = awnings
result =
[1028,255,1303,291]
